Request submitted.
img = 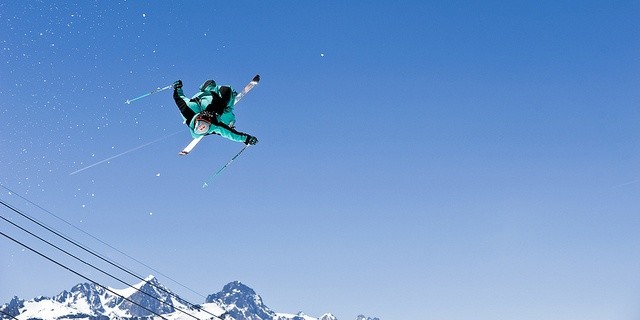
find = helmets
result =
[194,117,211,134]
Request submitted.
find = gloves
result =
[246,136,258,146]
[173,80,183,89]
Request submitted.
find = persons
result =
[173,80,258,146]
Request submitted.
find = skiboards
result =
[178,75,260,158]
